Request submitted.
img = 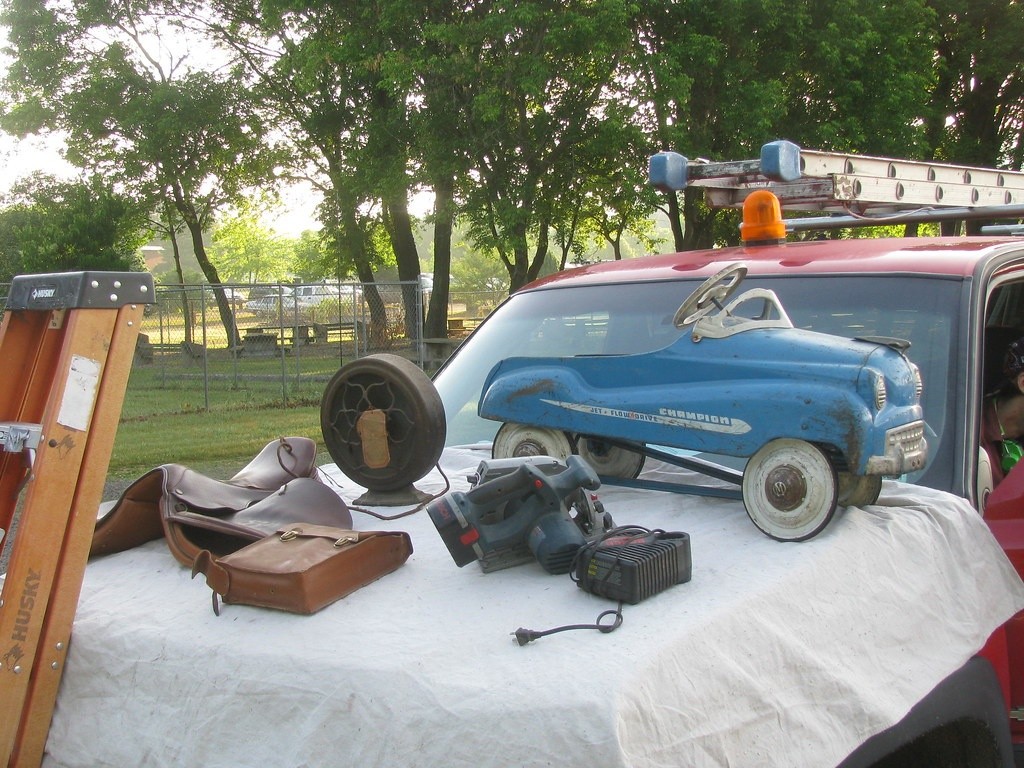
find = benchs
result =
[448,326,477,332]
[230,346,243,354]
[408,356,447,365]
[278,336,320,354]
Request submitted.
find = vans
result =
[248,287,293,303]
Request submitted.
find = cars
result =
[420,272,434,294]
[206,289,244,309]
[290,286,342,304]
[244,294,312,318]
[339,287,367,304]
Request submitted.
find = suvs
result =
[0,224,1024,768]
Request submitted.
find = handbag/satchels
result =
[191,520,414,615]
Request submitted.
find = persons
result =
[982,337,1024,476]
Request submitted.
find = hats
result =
[986,336,1024,395]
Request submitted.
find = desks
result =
[412,337,458,368]
[238,324,312,342]
[242,332,279,358]
[447,318,466,336]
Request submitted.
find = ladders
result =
[0,272,158,767]
